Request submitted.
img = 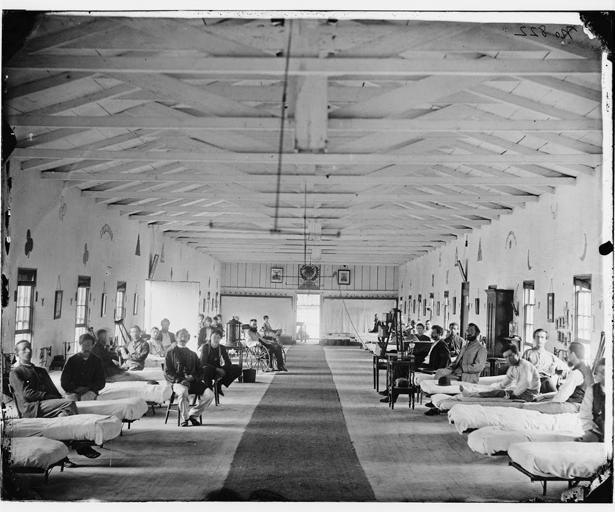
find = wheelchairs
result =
[244,332,287,373]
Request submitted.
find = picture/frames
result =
[546,292,555,324]
[270,267,284,284]
[100,293,108,317]
[422,298,427,316]
[53,289,63,320]
[337,269,351,286]
[475,297,480,315]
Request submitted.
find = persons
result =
[246,315,289,372]
[577,359,606,441]
[195,315,242,396]
[164,328,215,427]
[379,320,594,415]
[117,317,176,371]
[9,315,122,458]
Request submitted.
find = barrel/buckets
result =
[242,368,256,383]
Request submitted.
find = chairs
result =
[388,354,416,410]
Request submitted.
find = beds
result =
[0,327,233,484]
[430,393,581,408]
[363,324,512,374]
[421,378,570,393]
[467,427,601,457]
[413,373,508,386]
[447,404,590,434]
[507,441,609,495]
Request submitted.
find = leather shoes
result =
[425,402,434,407]
[214,383,224,396]
[182,420,188,426]
[192,420,199,425]
[379,390,388,396]
[76,446,100,459]
[425,409,440,416]
[380,397,396,403]
